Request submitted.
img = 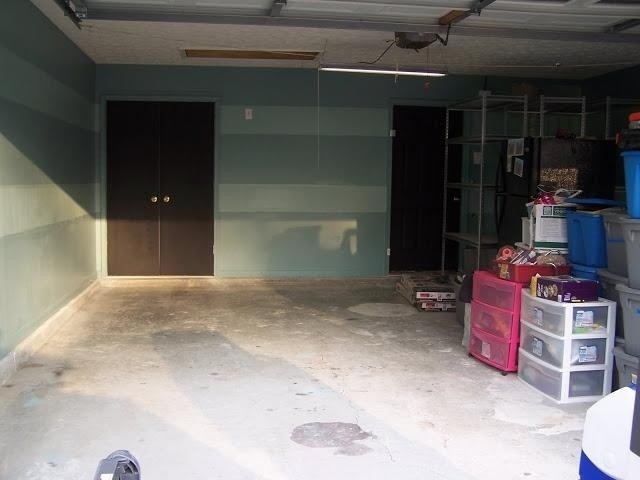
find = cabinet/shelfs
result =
[441,93,640,276]
[517,286,617,405]
[468,266,530,375]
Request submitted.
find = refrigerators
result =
[494,136,616,248]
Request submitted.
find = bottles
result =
[623,112,628,129]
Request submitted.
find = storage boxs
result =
[570,219,640,480]
